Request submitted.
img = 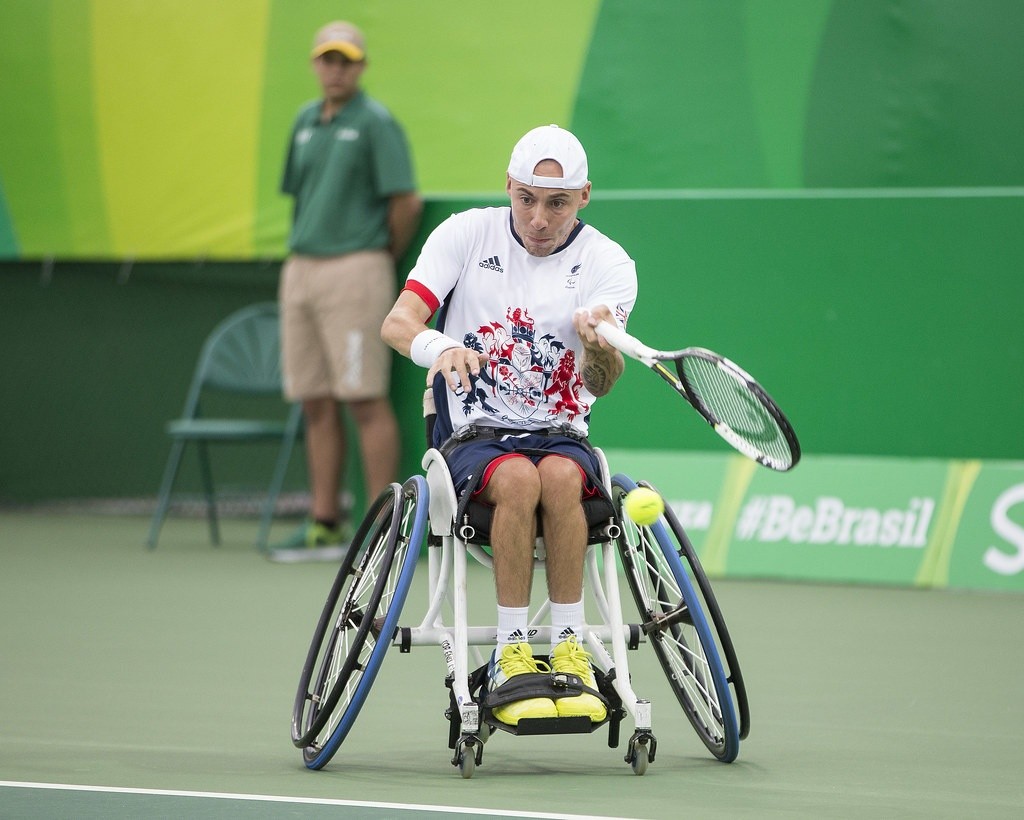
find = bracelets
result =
[410,329,466,369]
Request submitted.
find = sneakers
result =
[268,519,344,562]
[487,642,558,725]
[548,633,608,722]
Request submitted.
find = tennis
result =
[624,487,664,526]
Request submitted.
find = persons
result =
[265,21,419,567]
[378,124,639,724]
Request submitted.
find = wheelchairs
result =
[289,365,753,774]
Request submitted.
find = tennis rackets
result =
[578,310,806,474]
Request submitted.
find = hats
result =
[308,19,366,62]
[507,124,588,189]
